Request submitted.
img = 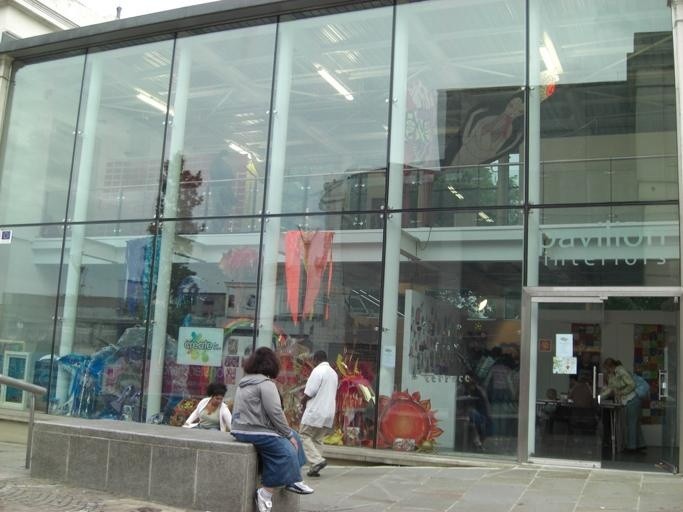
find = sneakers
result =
[254,490,272,512]
[621,446,646,452]
[286,481,314,494]
[308,460,327,476]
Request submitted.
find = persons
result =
[179,383,231,431]
[230,345,313,511]
[207,147,235,234]
[444,91,525,166]
[567,374,592,417]
[297,349,339,478]
[444,345,518,452]
[599,357,647,454]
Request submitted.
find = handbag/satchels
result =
[631,373,649,396]
